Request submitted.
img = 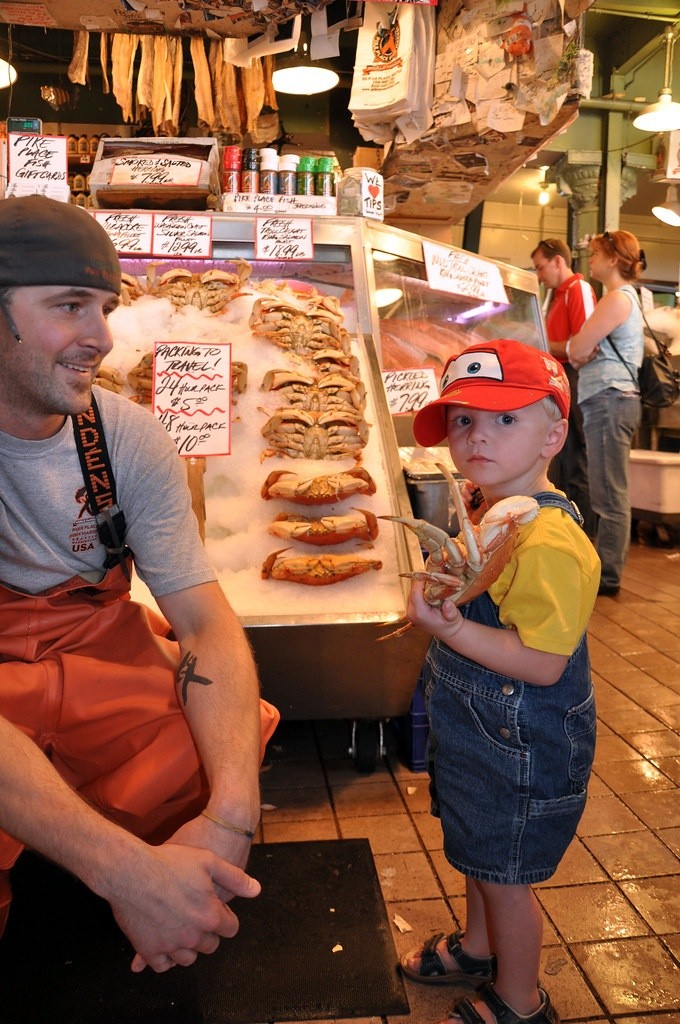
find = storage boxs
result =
[396,679,428,772]
[629,449,679,514]
[406,480,469,535]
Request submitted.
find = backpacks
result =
[604,289,680,408]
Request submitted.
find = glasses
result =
[538,240,564,257]
[603,231,615,249]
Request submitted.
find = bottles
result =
[337,167,385,222]
[58,133,98,153]
[67,170,93,209]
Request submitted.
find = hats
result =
[1,194,122,295]
[412,338,571,448]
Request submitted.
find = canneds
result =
[219,145,335,197]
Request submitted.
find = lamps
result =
[271,31,339,95]
[632,26,680,132]
[651,186,680,228]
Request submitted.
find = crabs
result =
[91,255,386,589]
[376,462,541,640]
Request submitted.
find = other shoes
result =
[598,585,622,597]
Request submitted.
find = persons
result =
[0,195,261,972]
[400,348,603,1024]
[569,230,642,596]
[531,239,599,542]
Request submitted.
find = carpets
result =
[0,838,412,1024]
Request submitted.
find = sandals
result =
[400,930,497,986]
[439,981,559,1024]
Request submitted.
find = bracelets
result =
[200,808,256,840]
